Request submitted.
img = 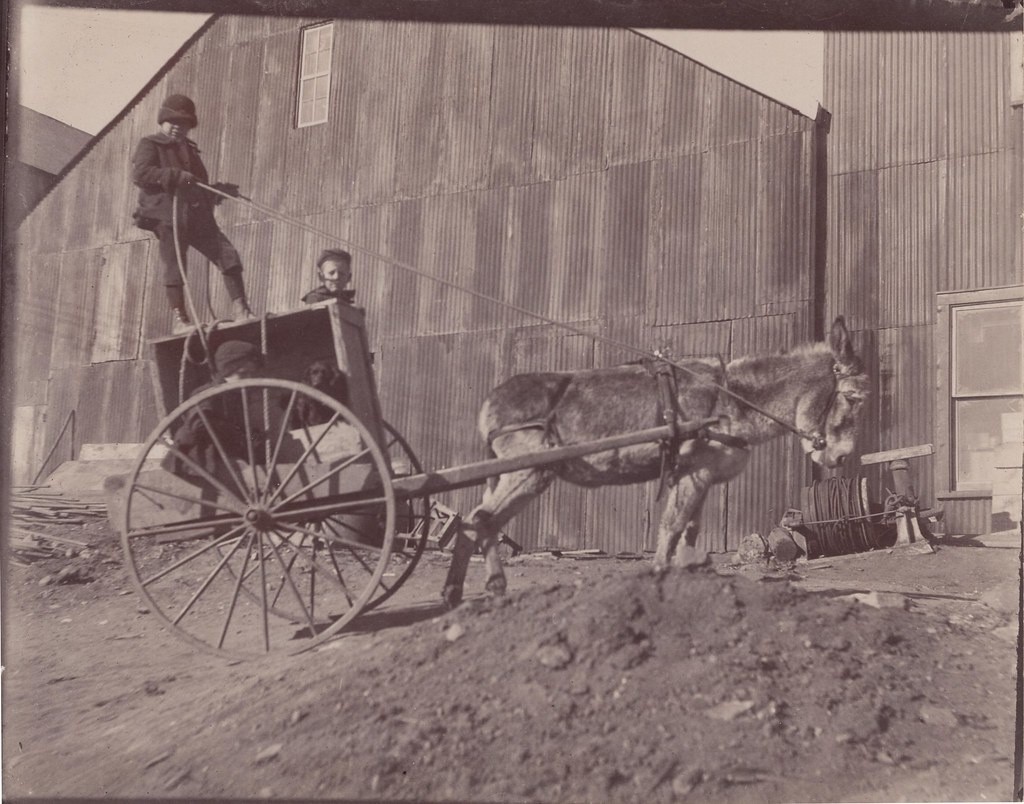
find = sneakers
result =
[171,309,204,335]
[234,298,262,321]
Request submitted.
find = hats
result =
[157,94,198,128]
[214,340,261,377]
[317,248,351,266]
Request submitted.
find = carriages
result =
[115,295,870,662]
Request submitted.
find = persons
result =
[302,248,357,306]
[132,94,259,335]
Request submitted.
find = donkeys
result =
[442,314,873,618]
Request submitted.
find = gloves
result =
[217,183,239,198]
[177,171,200,189]
